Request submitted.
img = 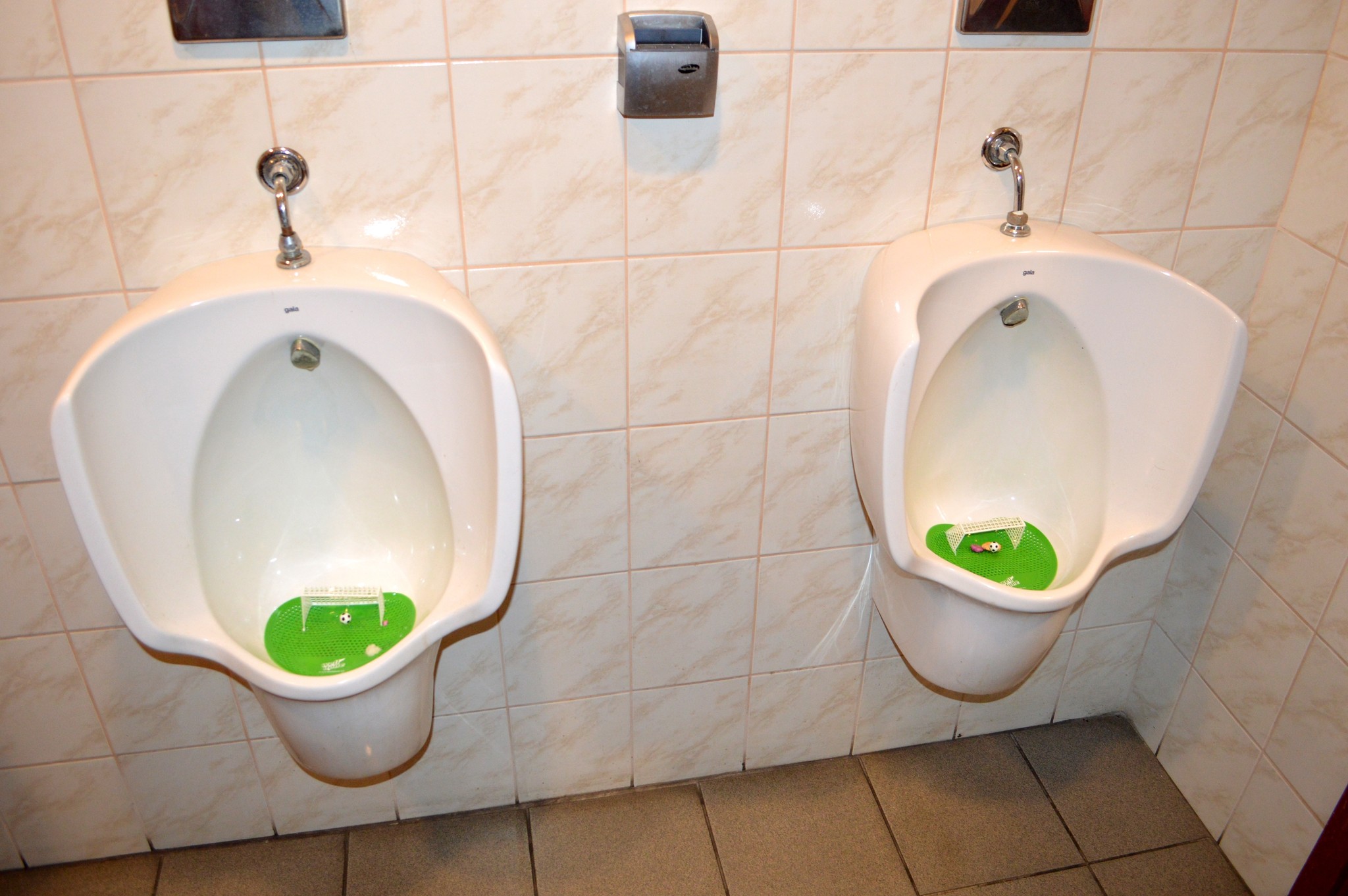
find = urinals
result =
[49,245,525,782]
[849,217,1248,696]
